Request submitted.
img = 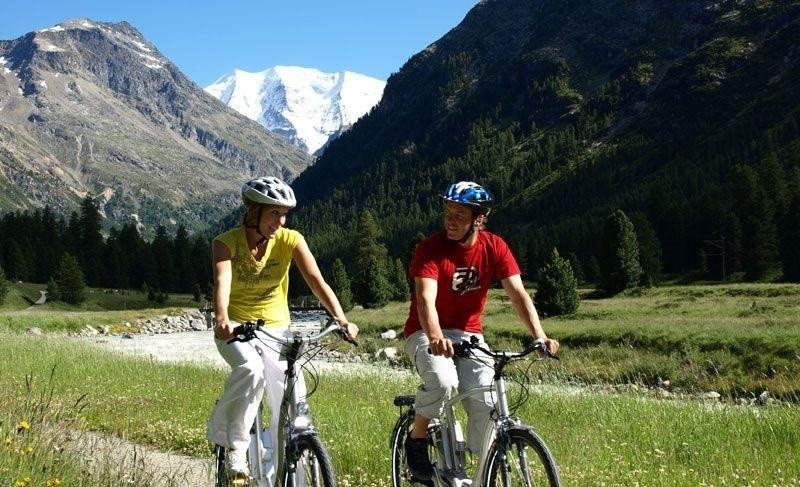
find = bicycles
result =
[210,317,361,487]
[387,334,565,487]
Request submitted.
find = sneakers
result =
[403,429,434,482]
[224,446,250,481]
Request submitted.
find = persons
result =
[401,179,562,483]
[203,174,361,486]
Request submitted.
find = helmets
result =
[241,175,298,210]
[437,180,494,217]
